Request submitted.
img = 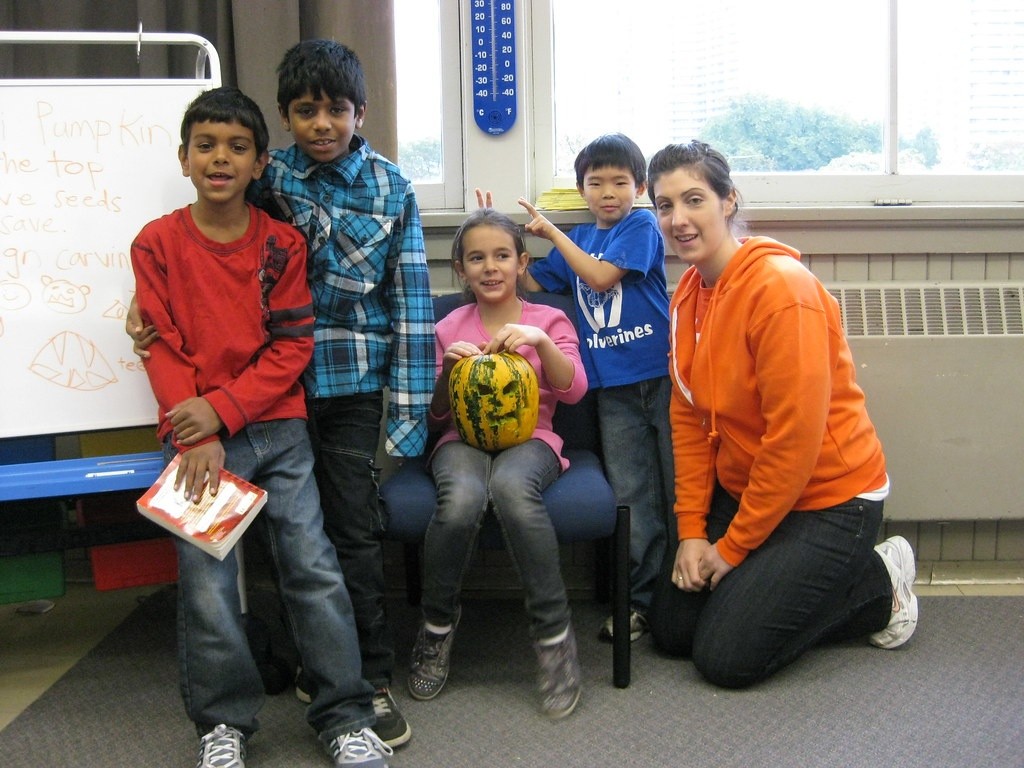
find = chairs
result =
[379,291,631,689]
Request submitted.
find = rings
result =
[676,576,682,582]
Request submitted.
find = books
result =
[136,452,268,561]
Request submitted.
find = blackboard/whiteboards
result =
[0,25,224,504]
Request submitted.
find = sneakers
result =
[297,670,311,703]
[196,723,247,768]
[369,687,411,748]
[323,727,393,768]
[535,627,580,720]
[598,609,648,643]
[407,607,462,700]
[869,536,918,649]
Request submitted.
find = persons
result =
[126,39,435,768]
[649,140,918,689]
[476,133,677,644]
[407,208,589,720]
